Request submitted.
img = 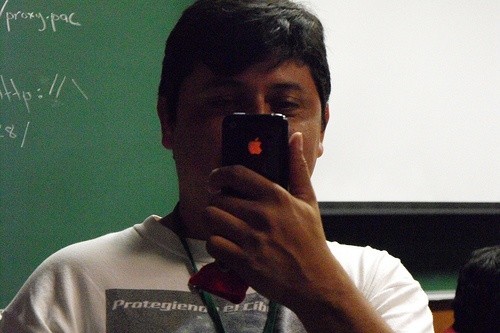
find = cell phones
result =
[222,111,289,197]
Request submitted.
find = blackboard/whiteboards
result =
[0,0,500,313]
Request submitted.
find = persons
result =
[0,0,436,333]
[452,245,500,333]
